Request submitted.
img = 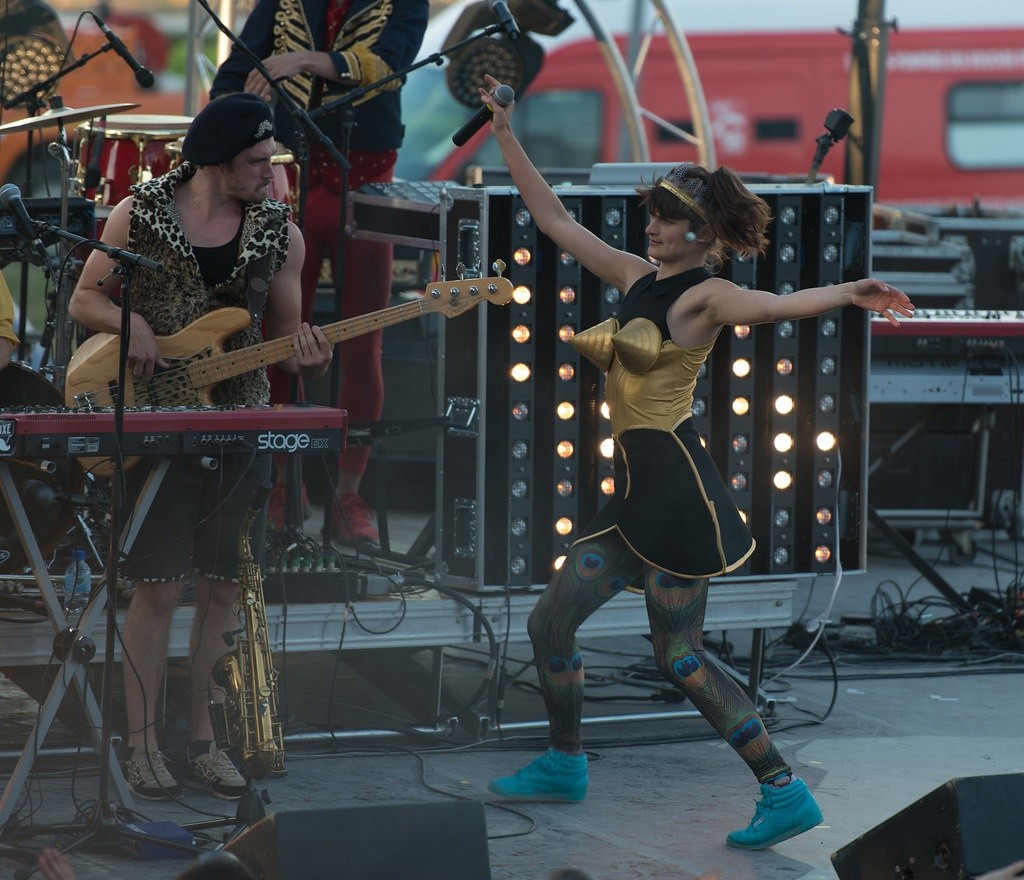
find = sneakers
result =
[268,479,313,530]
[181,740,248,800]
[119,738,183,801]
[486,746,589,800]
[725,777,824,850]
[321,491,380,557]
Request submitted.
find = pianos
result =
[867,305,1024,567]
[1,402,355,828]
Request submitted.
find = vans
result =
[392,1,1024,207]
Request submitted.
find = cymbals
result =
[0,102,143,135]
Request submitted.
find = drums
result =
[0,360,86,576]
[73,114,195,298]
[164,135,301,226]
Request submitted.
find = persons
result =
[37,848,250,880]
[479,73,916,853]
[208,0,430,539]
[0,93,334,800]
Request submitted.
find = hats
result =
[181,91,276,165]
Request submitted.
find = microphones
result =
[83,115,106,189]
[94,14,154,88]
[451,85,515,147]
[0,184,49,259]
[22,478,54,507]
[487,0,520,41]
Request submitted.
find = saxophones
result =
[212,481,289,778]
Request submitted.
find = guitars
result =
[64,258,514,478]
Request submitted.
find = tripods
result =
[0,220,212,880]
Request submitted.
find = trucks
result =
[1,25,217,199]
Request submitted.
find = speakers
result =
[223,800,493,880]
[830,773,1024,880]
